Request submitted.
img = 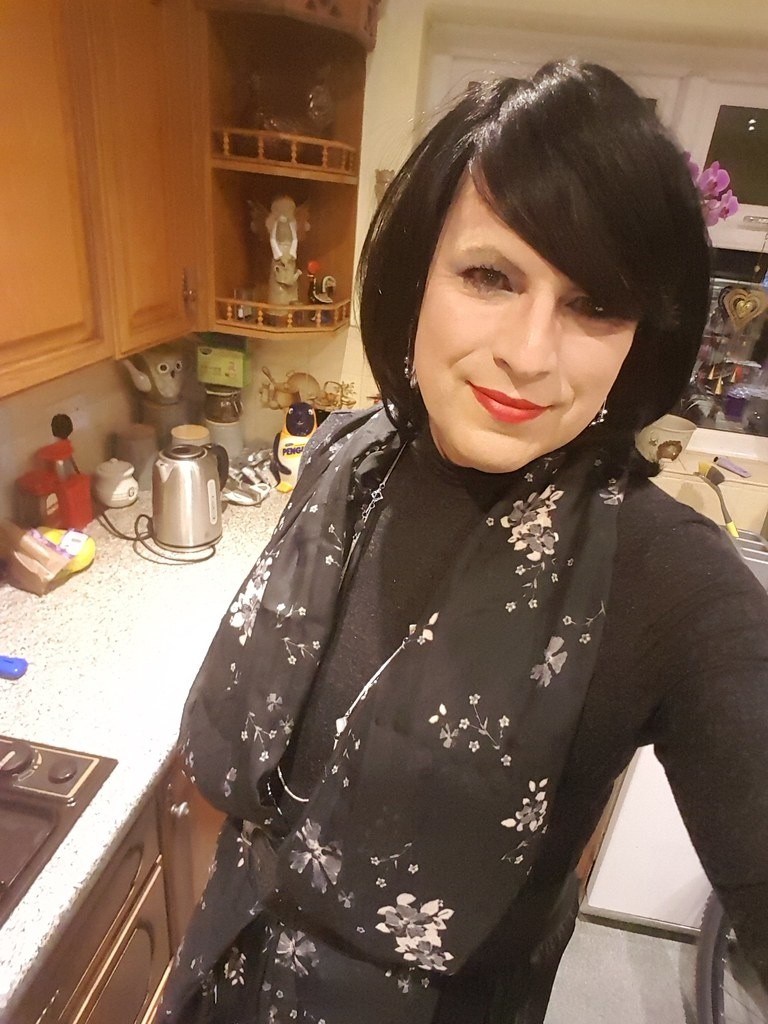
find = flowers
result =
[681,150,739,227]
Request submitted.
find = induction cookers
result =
[0,736,117,933]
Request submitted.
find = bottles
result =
[36,440,79,486]
[205,384,244,423]
[235,73,285,158]
[16,472,66,530]
[91,458,139,508]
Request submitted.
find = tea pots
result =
[274,263,302,287]
[120,346,194,405]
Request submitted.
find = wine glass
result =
[296,85,334,167]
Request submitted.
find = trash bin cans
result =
[718,524,768,597]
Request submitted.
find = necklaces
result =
[271,438,418,803]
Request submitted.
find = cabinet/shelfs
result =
[0,0,381,401]
[0,754,229,1024]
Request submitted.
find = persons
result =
[152,60,768,1024]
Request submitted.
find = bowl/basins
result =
[635,414,697,464]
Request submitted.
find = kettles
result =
[152,443,229,553]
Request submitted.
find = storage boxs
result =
[195,341,252,388]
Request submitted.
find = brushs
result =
[693,461,740,539]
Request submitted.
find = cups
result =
[204,417,243,458]
[114,423,159,492]
[143,396,186,450]
[171,424,210,448]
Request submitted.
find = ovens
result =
[8,777,197,1024]
